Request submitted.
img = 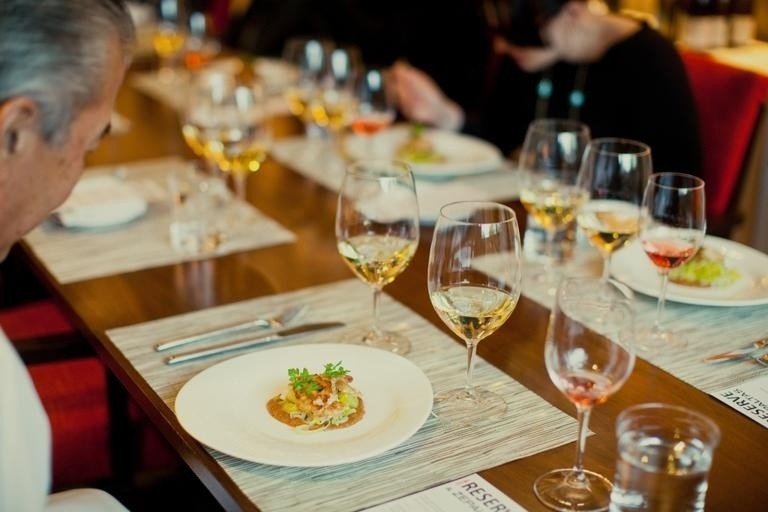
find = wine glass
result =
[620,175,706,354]
[572,139,653,288]
[310,48,358,158]
[426,199,524,425]
[518,120,588,269]
[534,276,636,512]
[185,69,236,185]
[183,9,217,77]
[335,157,420,354]
[349,68,395,156]
[153,0,183,86]
[284,37,329,162]
[207,89,272,231]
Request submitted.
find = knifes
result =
[163,322,344,365]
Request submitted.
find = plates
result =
[50,173,147,228]
[607,228,768,307]
[174,342,434,469]
[341,123,500,182]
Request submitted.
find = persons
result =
[0,0,136,511]
[380,0,702,228]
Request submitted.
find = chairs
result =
[677,47,768,237]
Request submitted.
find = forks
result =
[155,302,309,351]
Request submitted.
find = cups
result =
[609,401,721,512]
[169,160,231,255]
